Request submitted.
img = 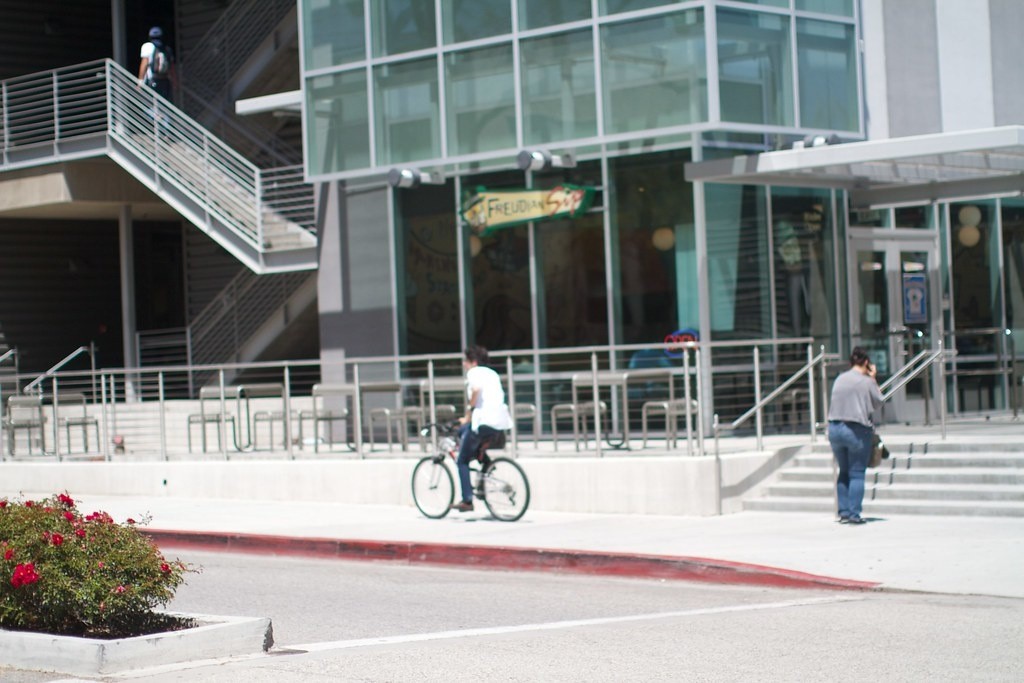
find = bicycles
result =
[411,417,531,522]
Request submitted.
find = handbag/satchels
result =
[869,433,889,466]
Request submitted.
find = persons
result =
[137,27,177,139]
[828,347,885,525]
[448,345,513,511]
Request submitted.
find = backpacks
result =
[149,41,169,75]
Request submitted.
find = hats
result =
[149,27,163,38]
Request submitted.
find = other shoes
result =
[479,464,495,474]
[841,513,866,524]
[452,502,473,511]
